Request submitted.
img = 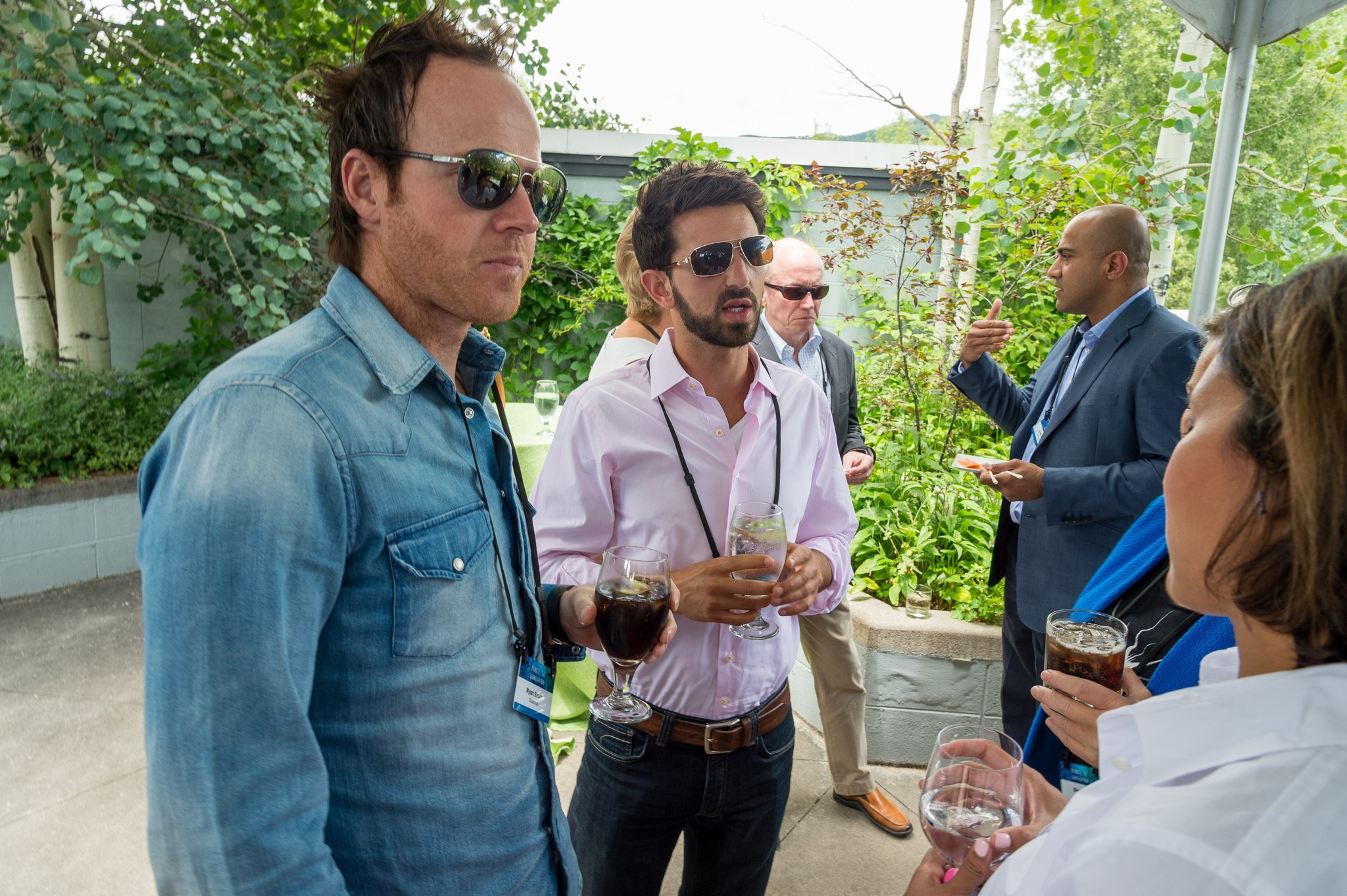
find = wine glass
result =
[727,501,788,640]
[586,545,672,724]
[534,380,560,438]
[919,724,1026,896]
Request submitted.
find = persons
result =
[528,157,857,896]
[748,239,914,837]
[136,5,684,896]
[585,206,672,381]
[902,257,1347,896]
[946,203,1231,793]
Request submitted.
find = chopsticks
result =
[988,470,1024,484]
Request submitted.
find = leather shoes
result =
[833,784,913,838]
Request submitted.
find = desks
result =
[502,402,563,500]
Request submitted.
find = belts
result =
[596,671,790,755]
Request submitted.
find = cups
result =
[905,584,933,619]
[1043,608,1129,710]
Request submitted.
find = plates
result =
[952,453,1008,473]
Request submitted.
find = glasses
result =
[659,235,775,277]
[379,148,568,224]
[764,282,829,300]
